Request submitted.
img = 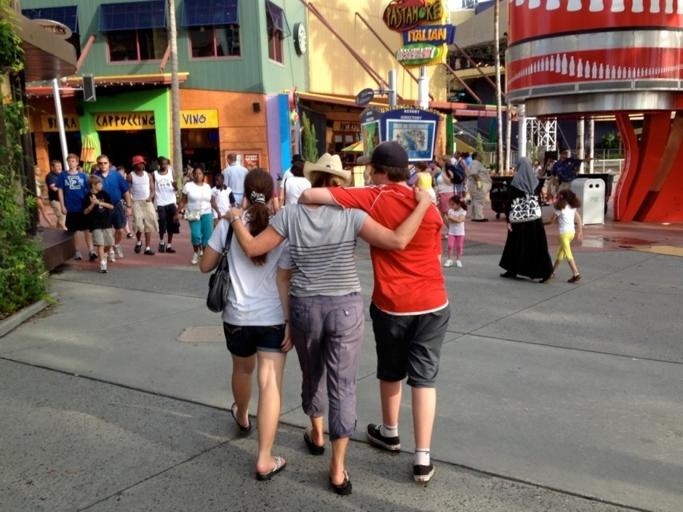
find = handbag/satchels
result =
[184,208,202,221]
[206,250,230,312]
[509,193,541,224]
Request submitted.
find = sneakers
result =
[74,232,203,273]
[367,424,401,450]
[456,259,462,267]
[500,271,583,283]
[444,259,453,267]
[413,462,436,483]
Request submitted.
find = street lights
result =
[52,74,97,170]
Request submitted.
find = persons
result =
[31,153,223,274]
[199,168,297,480]
[278,161,312,209]
[498,157,553,283]
[532,149,593,224]
[298,141,452,482]
[444,196,467,267]
[284,155,301,177]
[407,152,492,240]
[221,155,250,208]
[542,189,583,283]
[212,174,236,226]
[223,153,432,495]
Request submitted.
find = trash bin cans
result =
[570,178,606,226]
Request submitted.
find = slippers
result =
[256,455,286,481]
[231,401,252,434]
[303,427,325,455]
[329,469,352,495]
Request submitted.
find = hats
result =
[303,152,351,185]
[291,154,304,163]
[132,156,146,165]
[357,140,408,168]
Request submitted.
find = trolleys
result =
[577,173,613,214]
[489,175,547,222]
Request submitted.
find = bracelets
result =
[230,216,241,224]
[285,319,291,322]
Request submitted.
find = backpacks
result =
[445,163,464,184]
